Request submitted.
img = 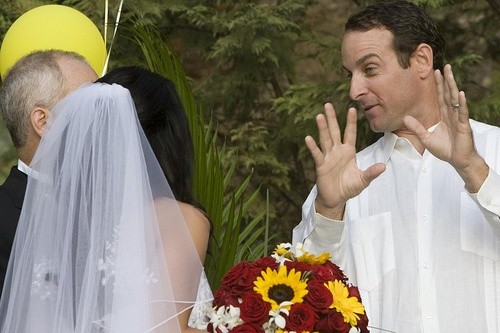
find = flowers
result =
[206,242,371,333]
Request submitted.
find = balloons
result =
[0,3,109,78]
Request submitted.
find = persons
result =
[291,0,500,333]
[0,65,212,333]
[0,48,99,332]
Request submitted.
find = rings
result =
[452,104,460,108]
